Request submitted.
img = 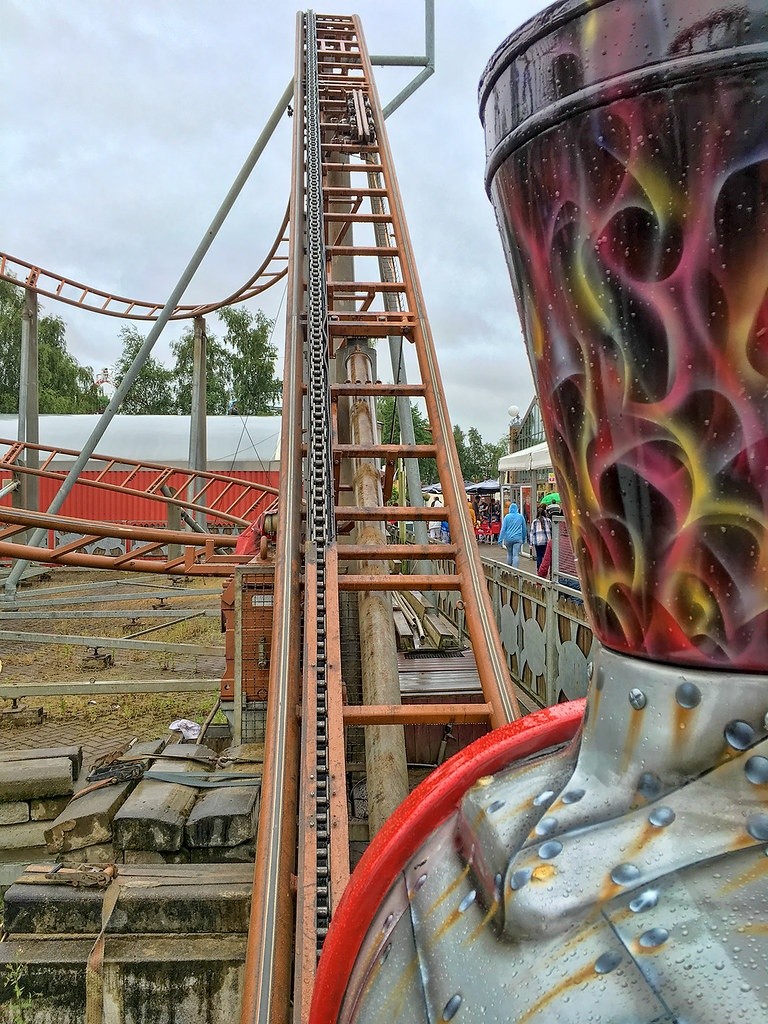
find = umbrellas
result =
[540,493,560,504]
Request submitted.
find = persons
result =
[472,496,481,515]
[530,508,552,572]
[498,503,526,568]
[429,497,450,543]
[390,503,398,527]
[489,499,496,513]
[538,539,552,580]
[547,499,561,518]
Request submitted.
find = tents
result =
[465,479,501,495]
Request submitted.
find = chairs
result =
[475,520,501,544]
